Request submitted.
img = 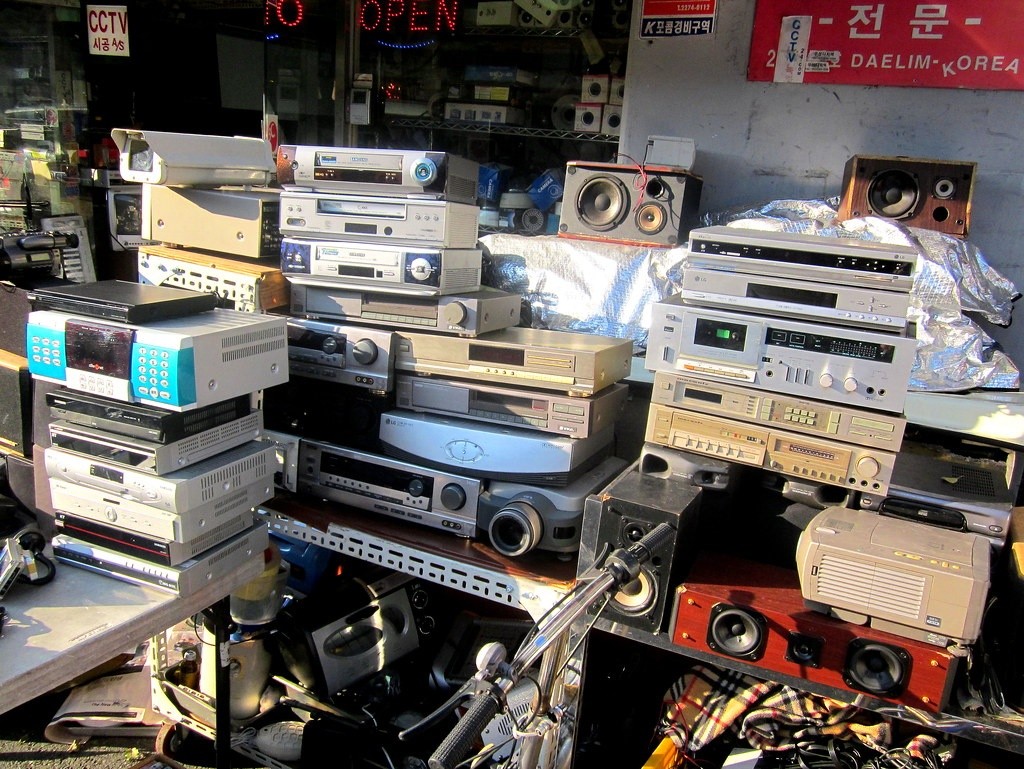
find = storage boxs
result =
[479,162,513,202]
[525,168,565,213]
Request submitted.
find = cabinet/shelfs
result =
[0,5,89,156]
[356,27,628,152]
[148,486,1024,769]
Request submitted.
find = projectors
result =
[795,504,992,650]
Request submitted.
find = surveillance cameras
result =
[111,127,279,187]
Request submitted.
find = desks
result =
[79,185,115,281]
[621,353,1024,502]
[1,538,265,769]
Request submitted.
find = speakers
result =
[571,470,704,636]
[667,546,959,717]
[556,161,702,249]
[837,155,978,239]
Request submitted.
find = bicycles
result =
[398,521,679,769]
